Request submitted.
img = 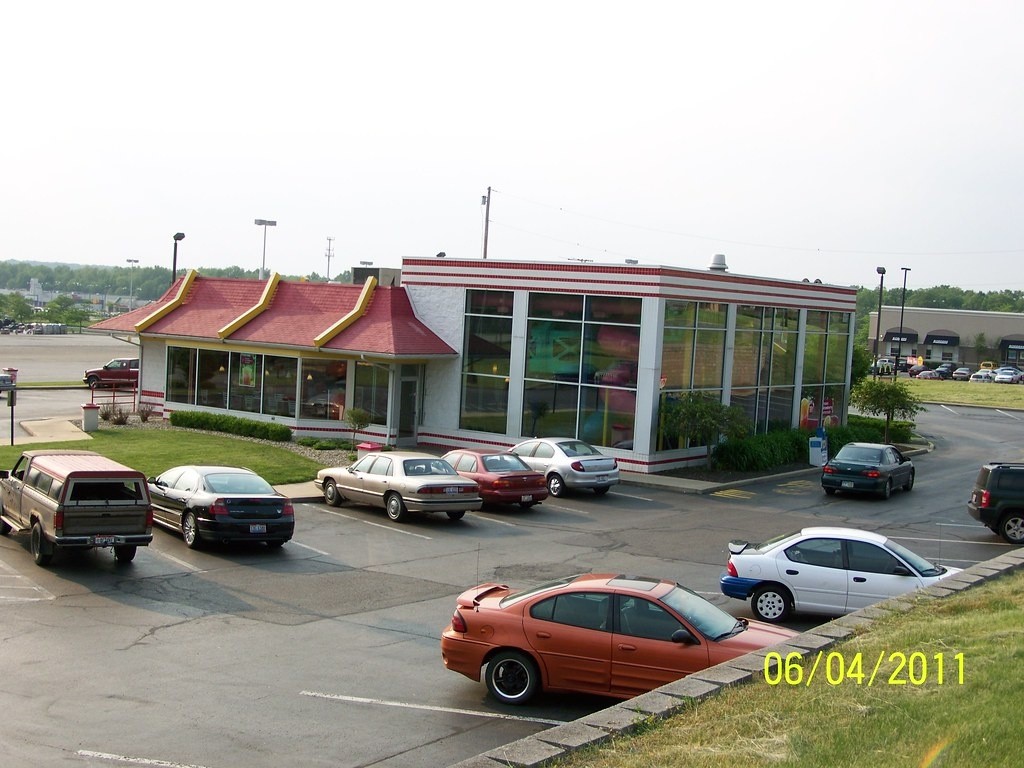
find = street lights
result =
[254,218,277,280]
[126,259,139,313]
[872,266,886,385]
[890,267,912,421]
[171,232,185,285]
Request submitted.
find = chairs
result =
[599,596,646,637]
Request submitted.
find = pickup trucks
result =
[0,449,155,567]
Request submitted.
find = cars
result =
[414,448,549,509]
[868,358,1024,384]
[438,572,804,708]
[819,440,916,501]
[484,436,621,498]
[145,463,296,552]
[306,394,341,419]
[313,450,484,523]
[718,525,969,626]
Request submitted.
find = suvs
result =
[965,461,1024,545]
[83,357,140,390]
